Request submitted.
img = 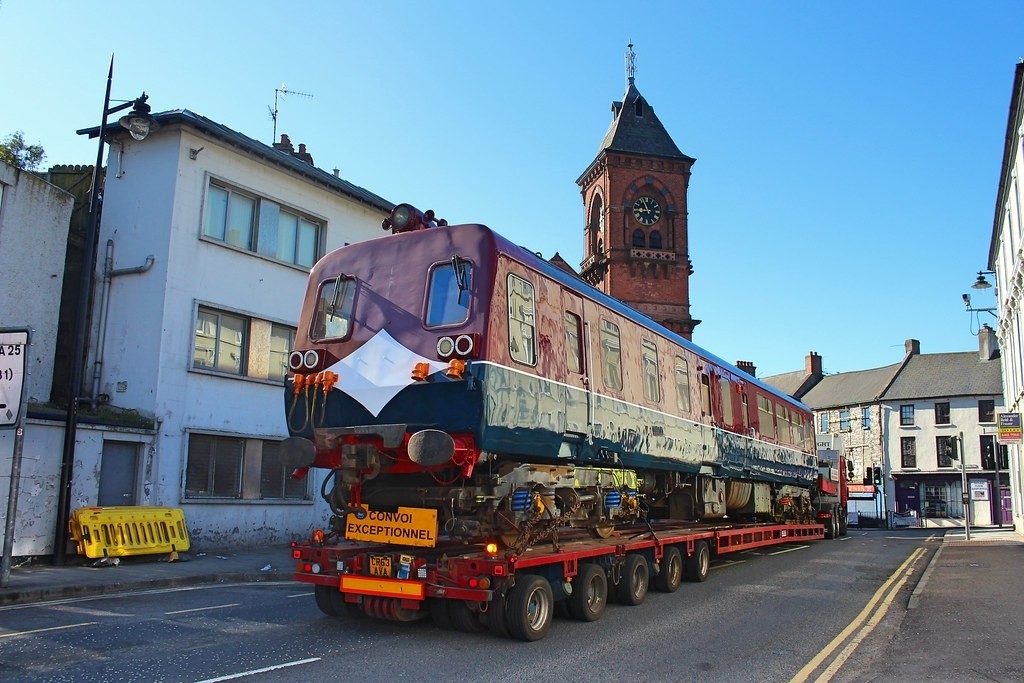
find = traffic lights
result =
[874,467,881,485]
[946,436,957,459]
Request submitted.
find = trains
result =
[285,201,818,552]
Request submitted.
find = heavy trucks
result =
[287,449,855,642]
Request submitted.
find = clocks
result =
[633,196,661,226]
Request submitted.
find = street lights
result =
[53,51,151,564]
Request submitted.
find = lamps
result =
[971,270,996,289]
